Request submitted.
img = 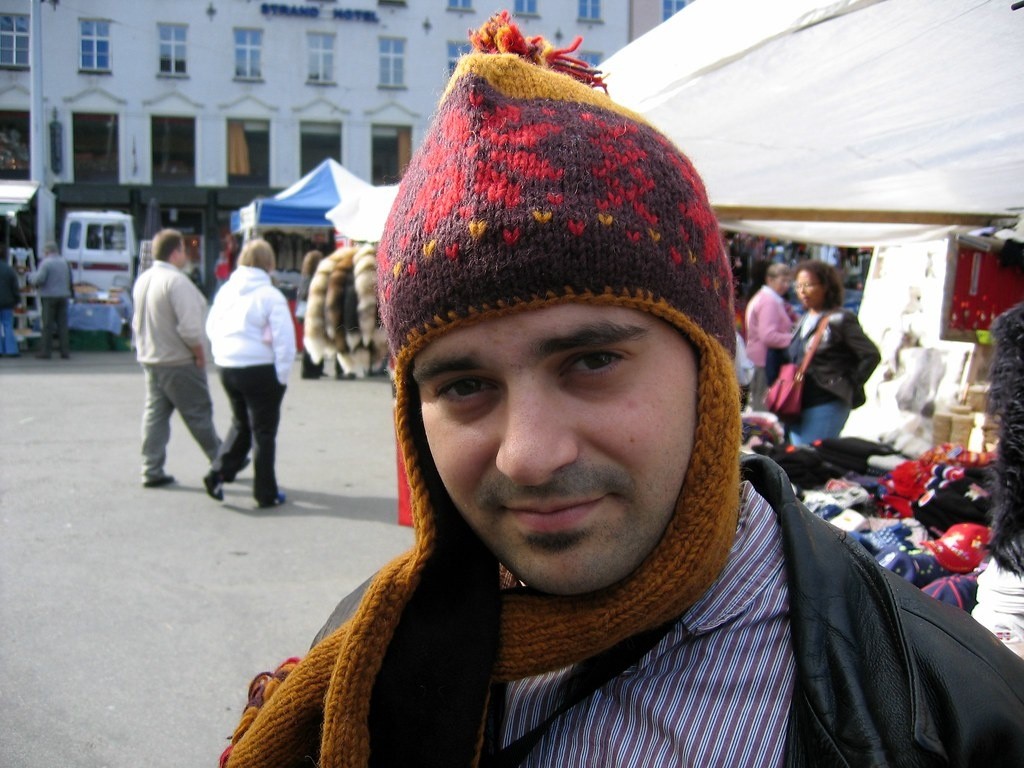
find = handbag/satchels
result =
[762,363,803,417]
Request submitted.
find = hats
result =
[216,10,741,768]
[760,437,1024,660]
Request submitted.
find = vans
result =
[60,209,137,300]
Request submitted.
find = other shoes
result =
[144,476,174,488]
[258,493,286,507]
[204,475,223,500]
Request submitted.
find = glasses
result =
[795,280,817,291]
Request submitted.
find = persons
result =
[216,11,1024,768]
[203,234,298,504]
[27,241,77,360]
[744,261,885,454]
[0,249,20,360]
[130,224,251,494]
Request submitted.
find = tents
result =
[0,180,44,267]
[543,2,1021,244]
[229,154,390,258]
